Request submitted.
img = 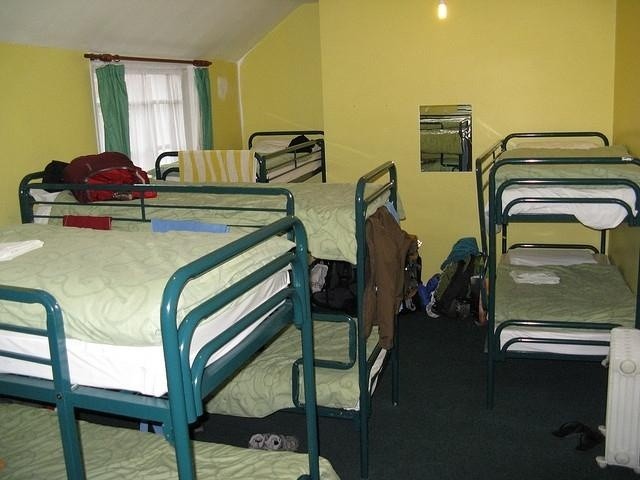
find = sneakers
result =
[250,432,298,450]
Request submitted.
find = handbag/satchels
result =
[66,152,157,202]
[431,237,480,316]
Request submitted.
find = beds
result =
[476,128,637,412]
[2,207,340,480]
[15,159,406,478]
[152,127,327,186]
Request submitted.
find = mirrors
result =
[419,105,475,172]
